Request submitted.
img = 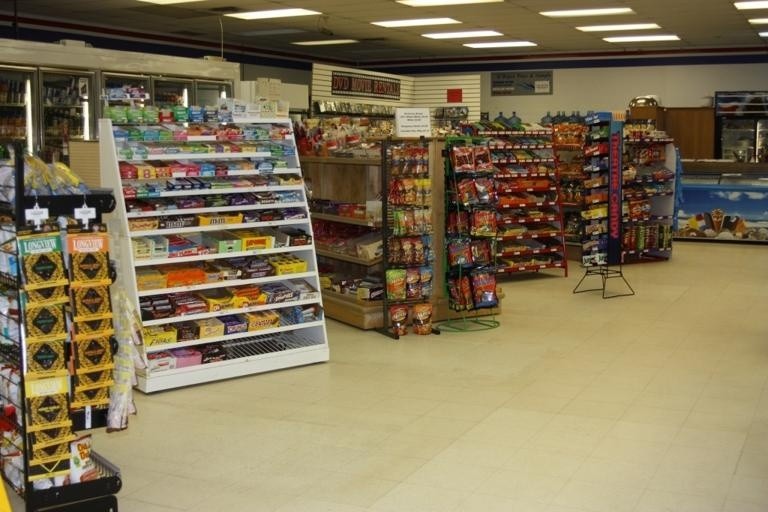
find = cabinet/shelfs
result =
[621,139,677,265]
[299,138,382,329]
[98,118,331,394]
[461,128,568,277]
[553,124,589,247]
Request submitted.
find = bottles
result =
[622,219,630,250]
[560,111,569,123]
[630,219,639,251]
[541,112,552,128]
[494,112,508,125]
[644,217,650,250]
[637,220,646,251]
[570,112,578,123]
[577,111,583,123]
[508,112,521,126]
[552,112,562,125]
[584,111,593,123]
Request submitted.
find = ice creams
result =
[711,209,723,233]
[726,216,747,234]
